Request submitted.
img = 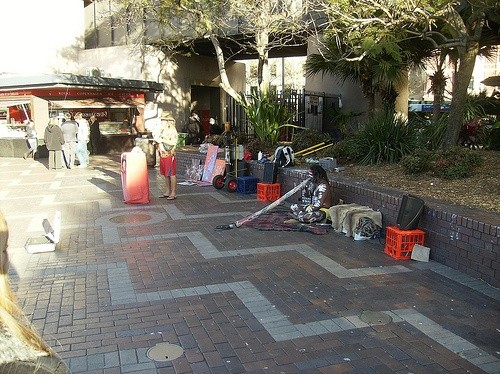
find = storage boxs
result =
[237,176,257,193]
[257,183,281,202]
[384,226,426,259]
[319,158,338,168]
[25,211,62,254]
[262,162,278,184]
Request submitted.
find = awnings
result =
[48,97,145,109]
[0,101,31,107]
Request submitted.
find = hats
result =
[160,112,175,121]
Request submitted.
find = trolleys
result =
[212,129,239,193]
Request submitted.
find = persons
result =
[23,119,39,161]
[187,115,224,145]
[87,115,101,155]
[288,164,333,222]
[44,112,91,167]
[157,112,179,200]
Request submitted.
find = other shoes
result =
[158,193,169,198]
[23,153,28,160]
[166,195,177,200]
[48,162,89,171]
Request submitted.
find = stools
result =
[330,204,380,237]
